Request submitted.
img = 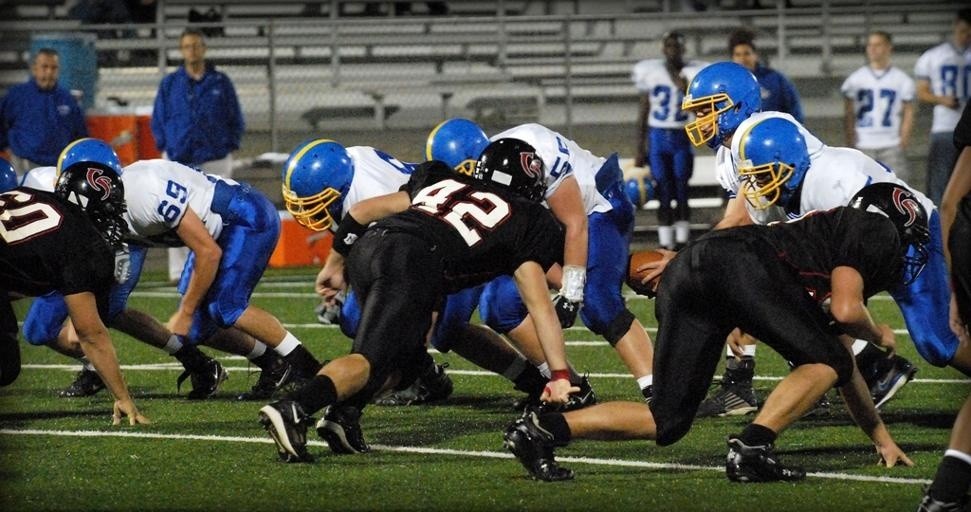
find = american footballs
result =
[627,250,662,297]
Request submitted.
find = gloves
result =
[552,296,578,329]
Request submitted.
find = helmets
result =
[424,118,488,177]
[736,118,810,210]
[475,138,547,203]
[849,183,931,286]
[282,138,355,231]
[0,138,128,252]
[680,62,763,150]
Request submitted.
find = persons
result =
[1,46,89,173]
[913,9,971,206]
[723,26,807,130]
[629,30,713,256]
[149,23,246,286]
[2,57,970,512]
[833,29,917,178]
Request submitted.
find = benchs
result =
[0,1,961,132]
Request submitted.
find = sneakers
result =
[502,412,575,482]
[641,384,652,404]
[726,433,806,484]
[237,361,298,402]
[375,362,454,404]
[177,357,229,400]
[509,377,550,414]
[799,394,831,421]
[315,404,368,455]
[696,378,758,419]
[861,356,918,408]
[61,368,105,398]
[918,484,971,512]
[270,361,324,401]
[561,369,596,413]
[257,400,315,463]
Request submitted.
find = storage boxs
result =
[82,103,164,167]
[268,207,335,269]
